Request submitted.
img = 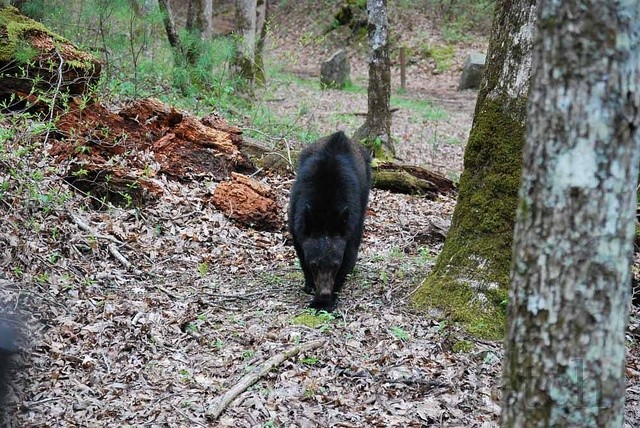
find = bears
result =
[287,128,371,316]
